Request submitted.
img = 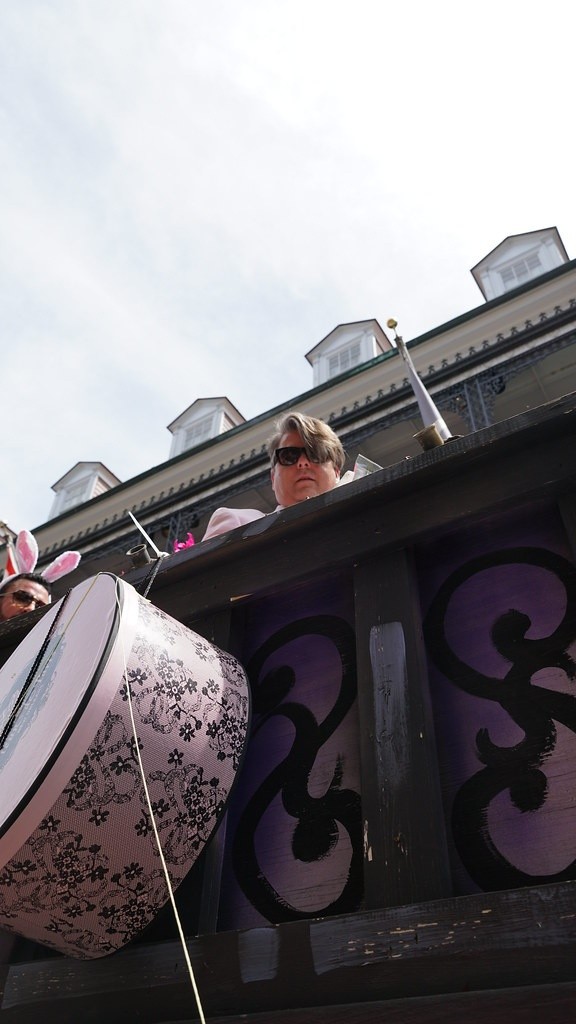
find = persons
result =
[201,411,373,543]
[0,571,52,625]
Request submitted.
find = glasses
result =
[0,590,46,611]
[273,446,328,466]
